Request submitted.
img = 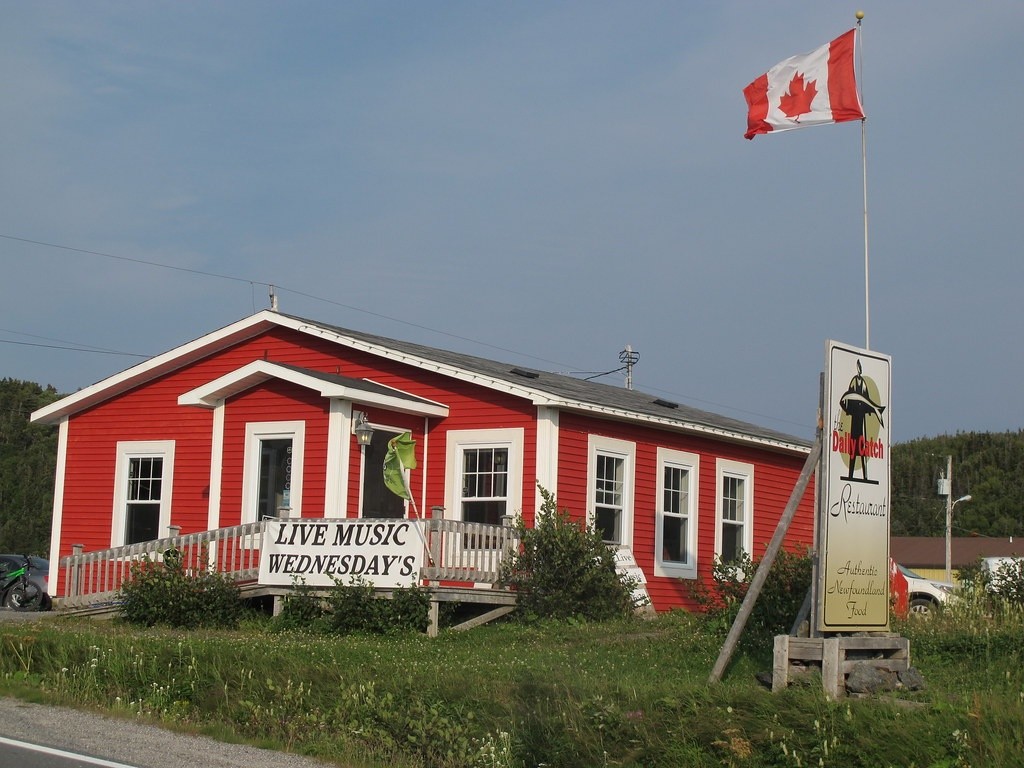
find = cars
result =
[898,562,963,621]
[1,555,49,595]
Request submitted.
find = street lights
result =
[945,494,972,588]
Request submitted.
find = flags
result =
[382,431,416,501]
[743,28,863,143]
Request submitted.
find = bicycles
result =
[0,553,43,612]
